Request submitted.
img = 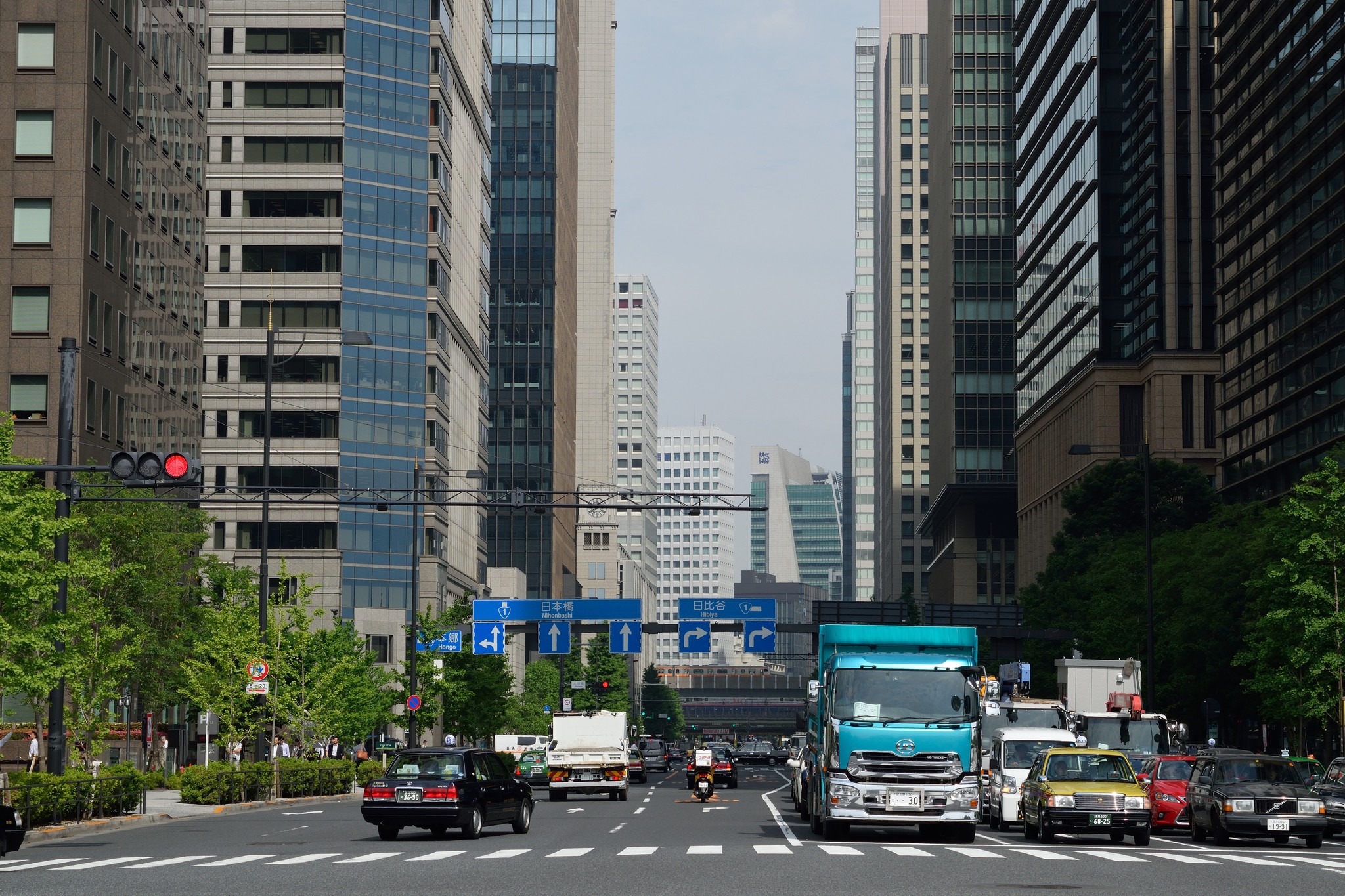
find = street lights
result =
[632,659,639,718]
[407,457,486,745]
[256,267,377,632]
[1067,443,1155,715]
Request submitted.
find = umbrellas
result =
[305,741,324,749]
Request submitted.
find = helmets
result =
[700,746,708,750]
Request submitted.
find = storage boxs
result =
[696,750,712,766]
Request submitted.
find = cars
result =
[1184,752,1328,851]
[686,746,738,789]
[977,659,1345,850]
[1017,736,1153,847]
[686,741,737,764]
[668,749,684,762]
[730,742,790,767]
[360,746,536,843]
[0,803,26,858]
[628,747,647,784]
[514,749,549,787]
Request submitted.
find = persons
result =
[278,736,291,759]
[397,739,402,749]
[325,736,335,757]
[328,736,345,760]
[463,740,468,747]
[160,736,168,748]
[1267,769,1279,784]
[267,736,283,762]
[227,735,242,764]
[26,731,40,773]
[350,738,367,780]
[632,740,637,748]
[1007,744,1033,766]
[422,740,428,748]
[1222,767,1234,783]
[403,740,408,749]
[690,738,695,750]
[1053,761,1068,779]
[663,738,674,770]
[291,737,324,762]
[695,738,699,748]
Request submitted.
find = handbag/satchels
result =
[357,745,368,760]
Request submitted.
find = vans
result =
[364,735,407,762]
[637,734,670,773]
[666,743,676,751]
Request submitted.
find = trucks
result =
[494,735,549,764]
[545,709,637,802]
[787,731,808,761]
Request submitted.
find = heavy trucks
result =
[791,622,1000,845]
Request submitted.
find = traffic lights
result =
[667,717,675,720]
[108,450,193,484]
[641,712,652,716]
[592,688,608,694]
[592,681,609,688]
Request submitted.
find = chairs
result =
[718,751,724,754]
[1098,761,1114,776]
[526,756,534,760]
[403,764,419,772]
[422,760,439,771]
[445,765,461,773]
[1089,765,1098,773]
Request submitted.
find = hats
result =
[297,741,302,745]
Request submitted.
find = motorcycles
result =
[688,757,718,803]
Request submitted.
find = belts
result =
[334,756,335,757]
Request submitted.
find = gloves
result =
[714,763,717,764]
[691,762,693,764]
